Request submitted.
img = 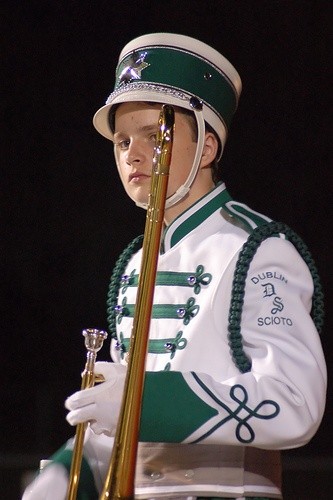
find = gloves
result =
[21,458,69,500]
[65,362,127,439]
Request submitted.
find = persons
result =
[21,33,327,500]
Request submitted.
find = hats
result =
[92,33,242,163]
